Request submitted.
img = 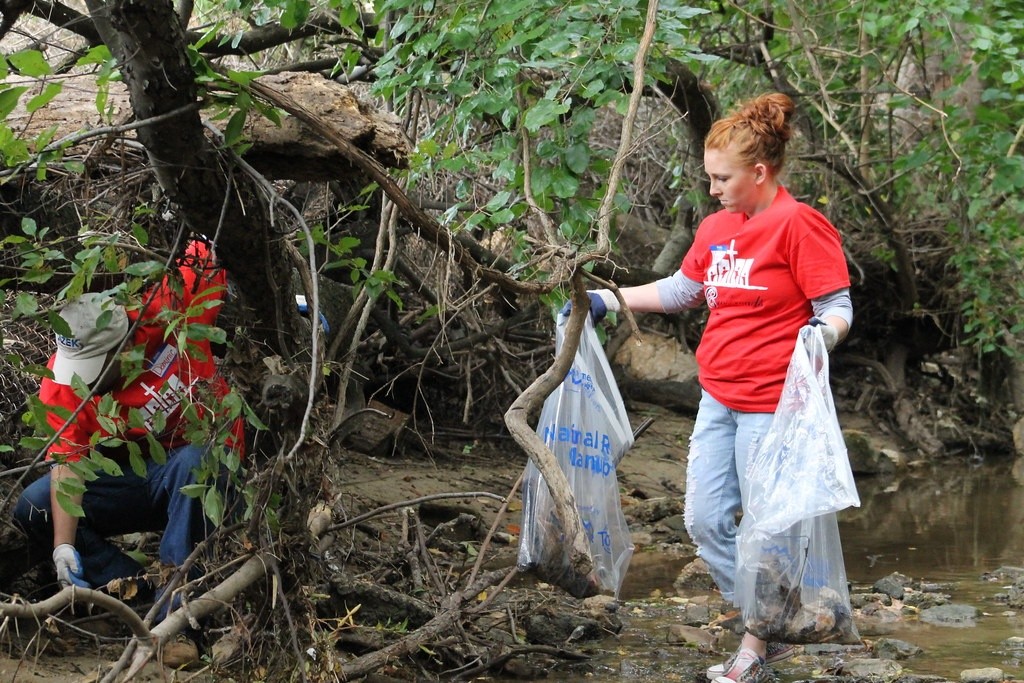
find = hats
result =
[52,293,128,385]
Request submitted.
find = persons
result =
[557,93,853,683]
[14,233,329,667]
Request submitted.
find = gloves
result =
[560,289,621,328]
[800,317,839,353]
[52,544,90,588]
[295,295,329,333]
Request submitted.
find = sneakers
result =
[706,649,769,683]
[163,633,199,670]
[706,641,794,679]
[80,620,115,637]
